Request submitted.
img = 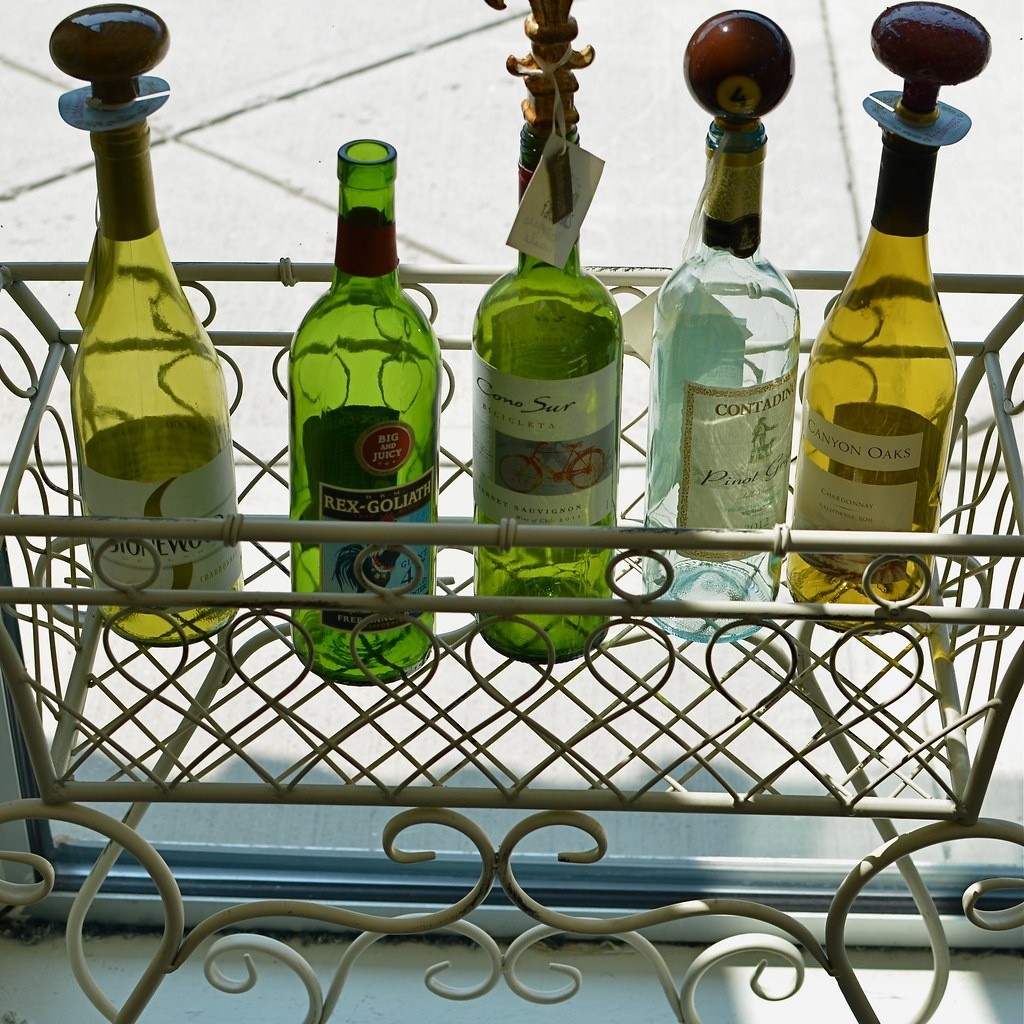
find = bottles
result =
[49,3,246,646]
[472,0,623,663]
[288,139,442,686]
[786,0,992,633]
[644,10,799,643]
